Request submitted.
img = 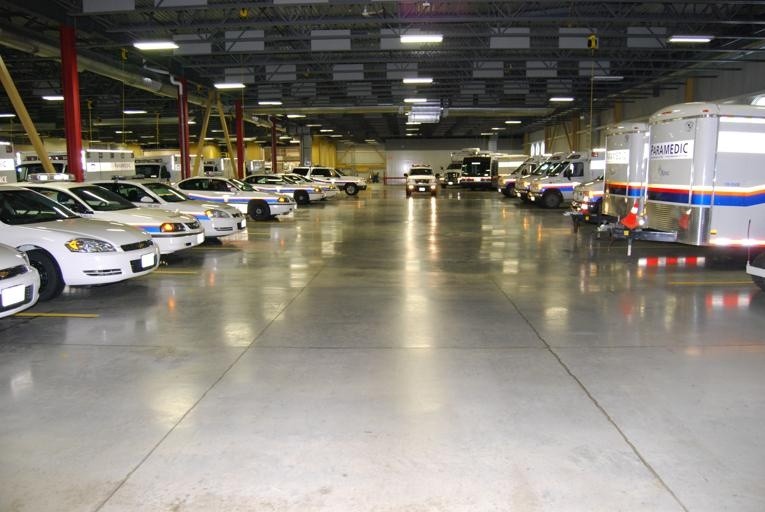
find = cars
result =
[1,183,163,301]
[6,178,205,258]
[1,243,44,322]
[89,171,341,243]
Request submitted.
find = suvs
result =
[290,166,369,196]
[403,163,441,197]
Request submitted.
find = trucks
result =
[459,147,606,211]
[203,156,247,178]
[133,151,207,182]
[245,158,267,175]
[439,148,469,189]
[11,143,140,184]
[597,120,651,229]
[602,89,765,286]
[265,161,272,174]
[567,173,612,225]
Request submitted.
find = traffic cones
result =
[580,192,591,214]
[677,208,694,229]
[618,199,643,230]
